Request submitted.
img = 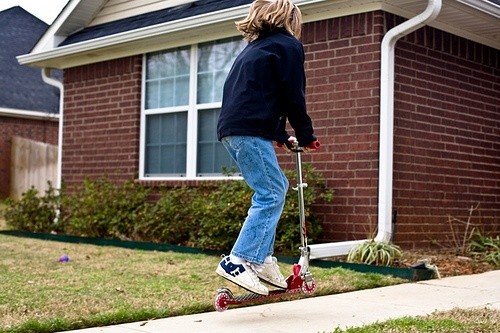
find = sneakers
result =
[215,256,269,296]
[251,257,288,289]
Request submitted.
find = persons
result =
[218,0,319,295]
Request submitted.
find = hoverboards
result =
[212,140,320,312]
